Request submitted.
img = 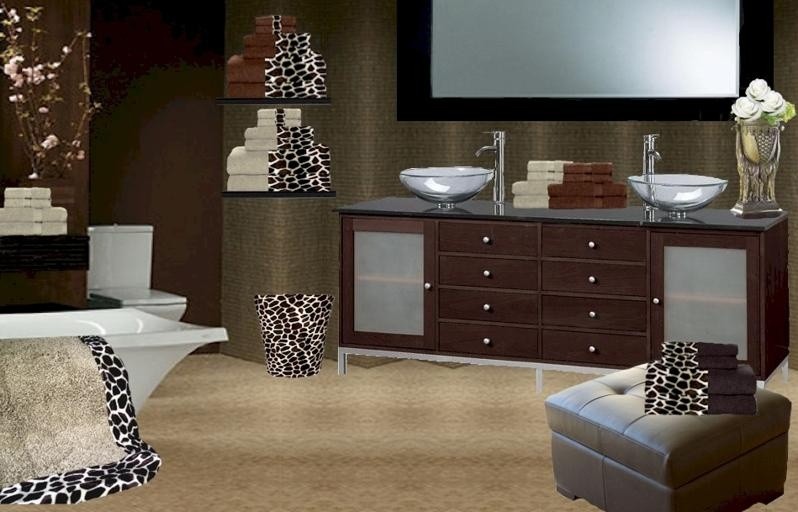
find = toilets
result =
[88,225,187,323]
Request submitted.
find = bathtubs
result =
[0,308,229,421]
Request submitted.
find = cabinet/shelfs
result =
[340,215,435,359]
[216,96,339,199]
[539,220,652,371]
[650,226,761,379]
[437,217,541,363]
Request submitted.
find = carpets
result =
[0,335,161,508]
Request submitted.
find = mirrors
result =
[395,1,774,120]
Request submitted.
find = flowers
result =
[0,0,111,179]
[728,77,797,128]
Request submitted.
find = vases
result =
[729,121,786,218]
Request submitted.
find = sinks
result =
[399,166,495,203]
[628,174,728,209]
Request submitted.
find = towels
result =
[640,338,763,414]
[511,157,628,210]
[0,186,68,238]
[226,12,335,191]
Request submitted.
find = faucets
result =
[643,133,661,174]
[475,130,505,203]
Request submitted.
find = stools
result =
[541,363,791,512]
